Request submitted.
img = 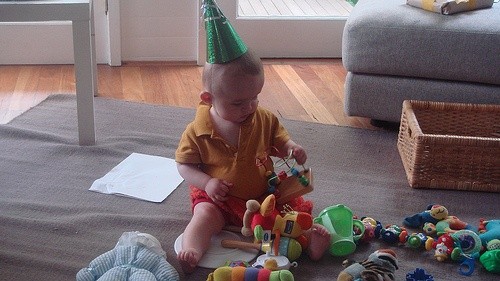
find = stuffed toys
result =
[337,249,399,281]
[241,195,312,242]
[431,203,480,233]
[76,231,180,281]
[206,260,293,281]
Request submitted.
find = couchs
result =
[342,0,500,125]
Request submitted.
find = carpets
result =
[0,95,497,281]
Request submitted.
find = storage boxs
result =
[396,99,500,191]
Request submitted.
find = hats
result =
[200,0,249,64]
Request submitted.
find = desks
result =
[0,0,98,145]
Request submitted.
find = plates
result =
[174,226,260,268]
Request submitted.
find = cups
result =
[314,204,365,256]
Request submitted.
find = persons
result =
[176,0,330,275]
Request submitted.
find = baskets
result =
[395,99,500,192]
